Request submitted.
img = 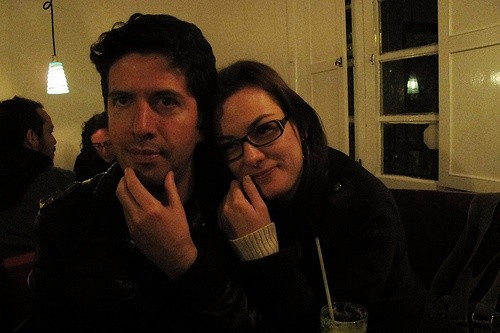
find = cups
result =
[321,303,367,333]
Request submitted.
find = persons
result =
[31,13,262,333]
[203,60,442,333]
[0,95,117,333]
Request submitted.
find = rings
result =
[128,240,136,249]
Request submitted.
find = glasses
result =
[90,140,112,151]
[213,115,288,164]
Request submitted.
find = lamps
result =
[43,0,70,95]
